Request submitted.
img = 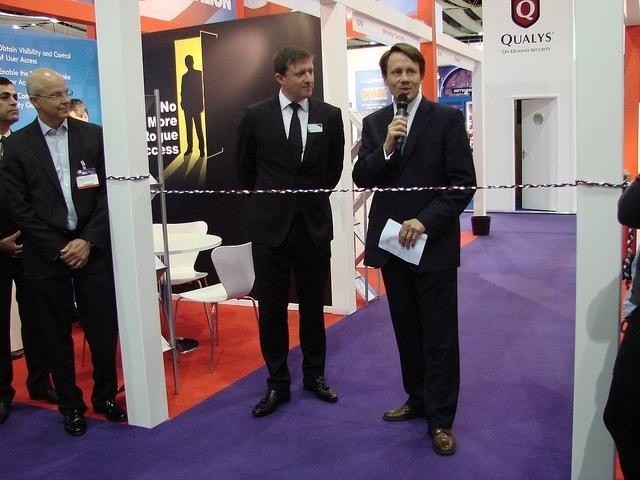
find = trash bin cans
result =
[470,216,492,236]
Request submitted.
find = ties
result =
[285,100,304,165]
[397,112,408,158]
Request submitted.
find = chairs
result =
[79,220,260,373]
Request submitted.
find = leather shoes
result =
[28,385,59,404]
[301,374,339,403]
[0,393,14,424]
[60,406,88,436]
[382,401,429,421]
[92,398,127,424]
[427,419,457,457]
[251,385,291,416]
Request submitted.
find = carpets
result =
[0,211,577,480]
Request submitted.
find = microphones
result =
[395,93,408,151]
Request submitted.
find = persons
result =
[3,68,127,436]
[0,77,58,426]
[602,174,640,480]
[352,42,477,456]
[68,98,89,123]
[180,54,205,157]
[236,45,345,417]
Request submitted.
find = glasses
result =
[36,90,75,99]
